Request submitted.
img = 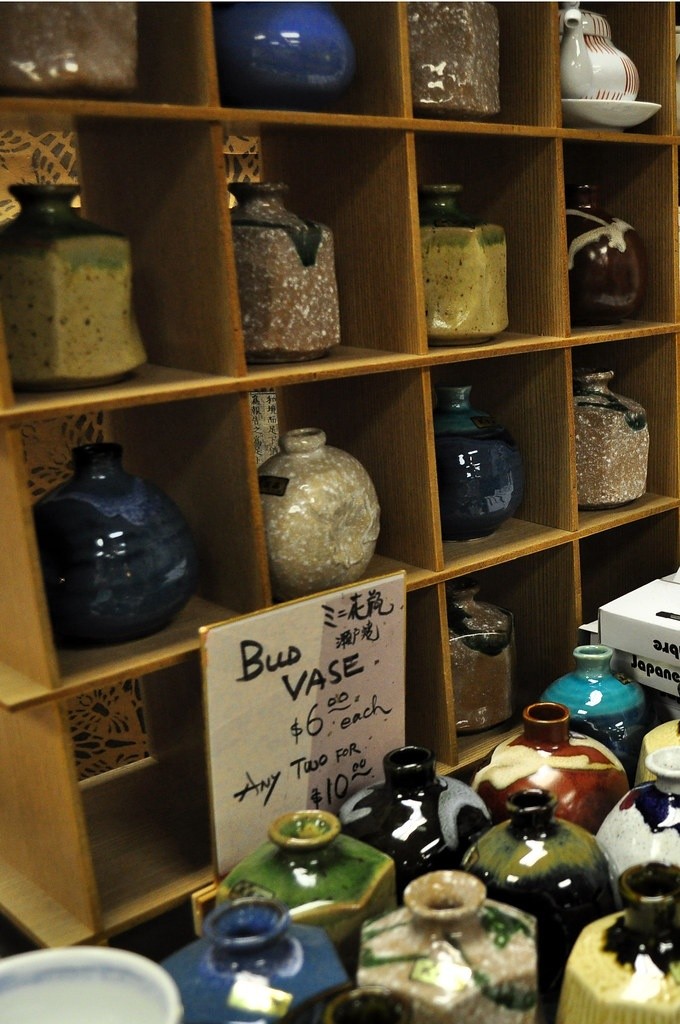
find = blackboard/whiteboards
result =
[197,569,409,879]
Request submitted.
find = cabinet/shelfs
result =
[0,3,680,949]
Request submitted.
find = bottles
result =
[214,3,355,106]
[573,364,648,511]
[418,183,511,347]
[0,185,150,390]
[256,428,382,604]
[153,583,679,1024]
[230,183,343,366]
[405,0,504,119]
[1,1,138,95]
[29,440,198,642]
[434,382,525,540]
[563,185,649,325]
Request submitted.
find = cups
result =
[0,945,184,1024]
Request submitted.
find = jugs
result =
[560,2,641,100]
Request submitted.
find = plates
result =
[560,100,663,133]
